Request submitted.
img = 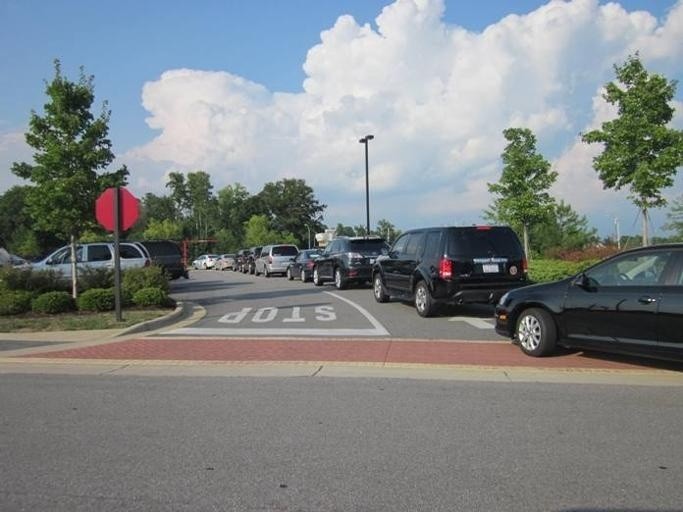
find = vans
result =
[140,239,183,281]
[11,241,152,281]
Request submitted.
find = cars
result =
[192,253,236,271]
[286,249,324,282]
[0,246,25,266]
[493,242,683,370]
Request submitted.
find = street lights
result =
[359,135,374,236]
[302,215,323,249]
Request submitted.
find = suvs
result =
[310,236,391,289]
[371,224,529,318]
[233,244,299,277]
